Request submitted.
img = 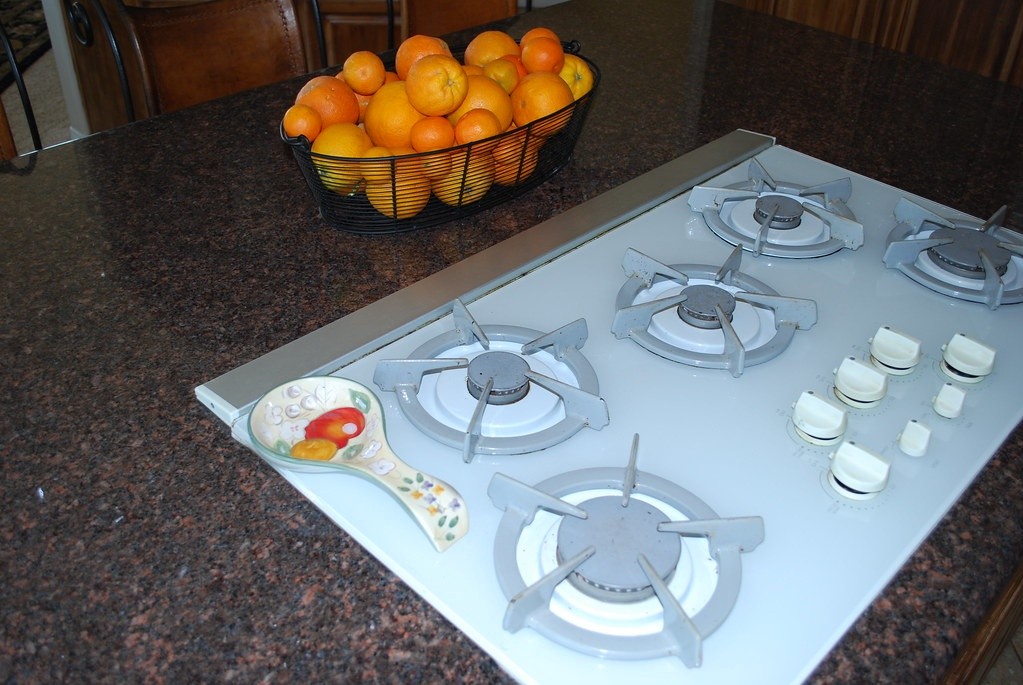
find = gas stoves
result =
[195,129,1023,685]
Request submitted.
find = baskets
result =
[279,35,601,236]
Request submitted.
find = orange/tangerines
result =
[282,29,594,220]
